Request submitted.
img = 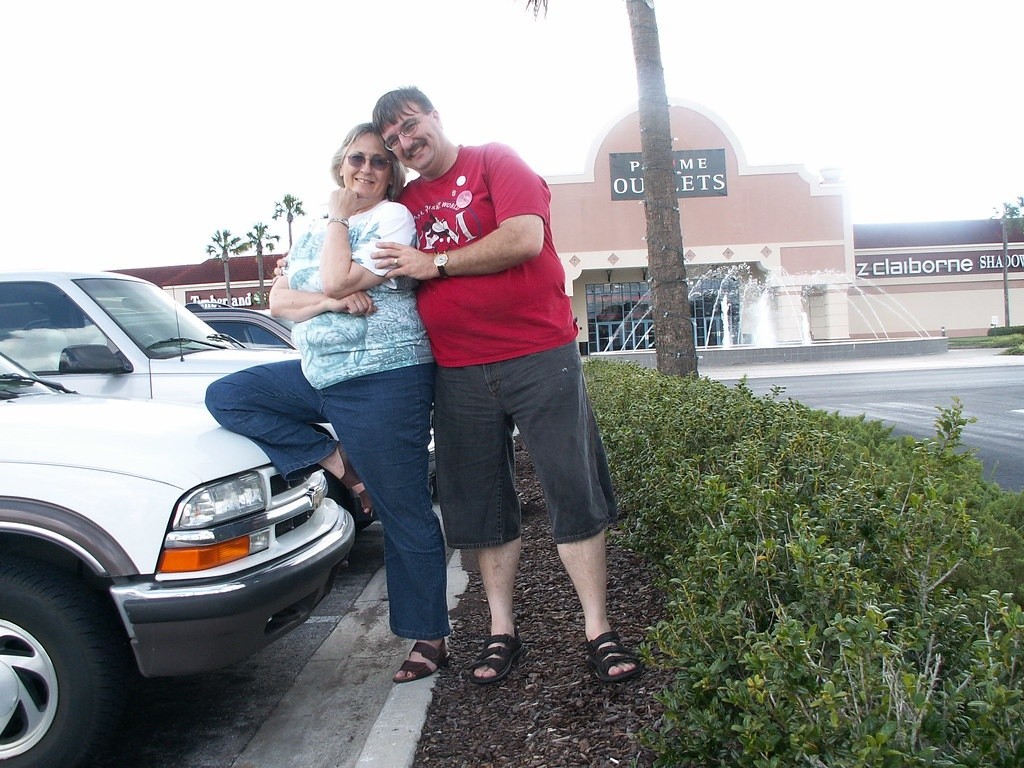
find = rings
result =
[394,258,399,266]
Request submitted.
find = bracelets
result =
[327,217,349,228]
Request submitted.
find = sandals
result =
[469,628,524,683]
[585,631,644,683]
[393,637,450,683]
[337,441,374,517]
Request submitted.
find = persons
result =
[206,122,451,684]
[372,86,646,683]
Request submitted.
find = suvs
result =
[0,273,438,536]
[99,304,298,352]
[0,351,357,768]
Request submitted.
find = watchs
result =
[433,254,449,278]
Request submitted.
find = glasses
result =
[345,151,392,170]
[384,114,430,150]
